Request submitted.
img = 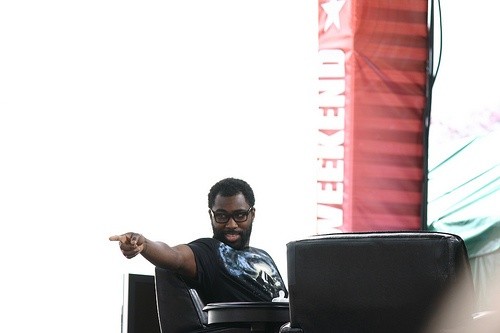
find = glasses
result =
[210,206,252,223]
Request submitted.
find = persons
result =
[109,178,289,306]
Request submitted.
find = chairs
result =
[279,231,500,333]
[155,263,206,333]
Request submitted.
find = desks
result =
[202,302,290,333]
